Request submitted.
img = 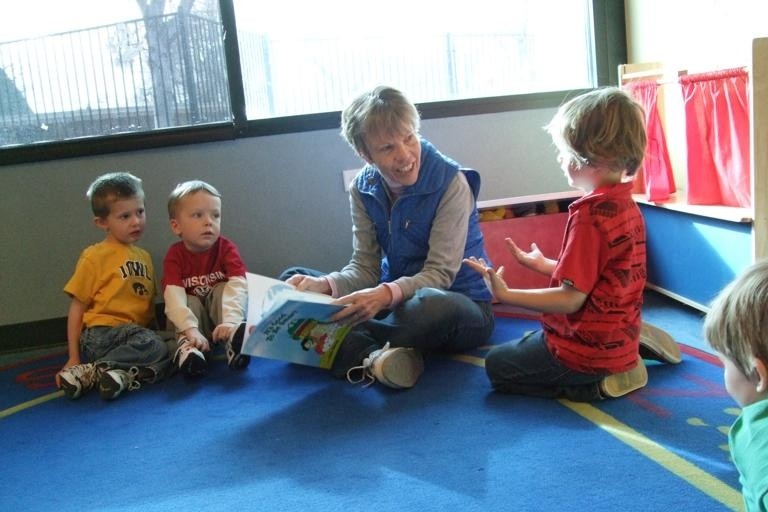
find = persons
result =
[160,179,253,379]
[279,85,495,390]
[701,256,768,512]
[462,86,683,403]
[55,171,178,401]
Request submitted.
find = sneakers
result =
[346,340,425,391]
[172,341,208,389]
[58,363,100,400]
[95,364,142,401]
[225,321,250,371]
[636,320,682,366]
[589,353,648,403]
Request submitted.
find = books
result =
[240,270,356,369]
